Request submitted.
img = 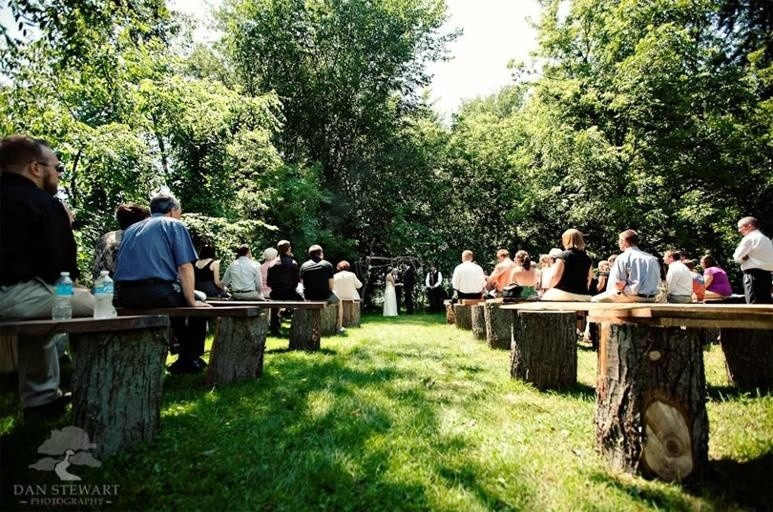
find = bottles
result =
[50,270,72,323]
[91,269,115,320]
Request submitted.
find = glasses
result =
[30,161,63,173]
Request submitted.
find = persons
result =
[0,137,363,424]
[383,227,732,342]
[732,216,773,303]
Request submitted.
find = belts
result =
[235,291,251,293]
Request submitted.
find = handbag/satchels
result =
[503,283,522,297]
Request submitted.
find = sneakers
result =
[24,393,72,420]
